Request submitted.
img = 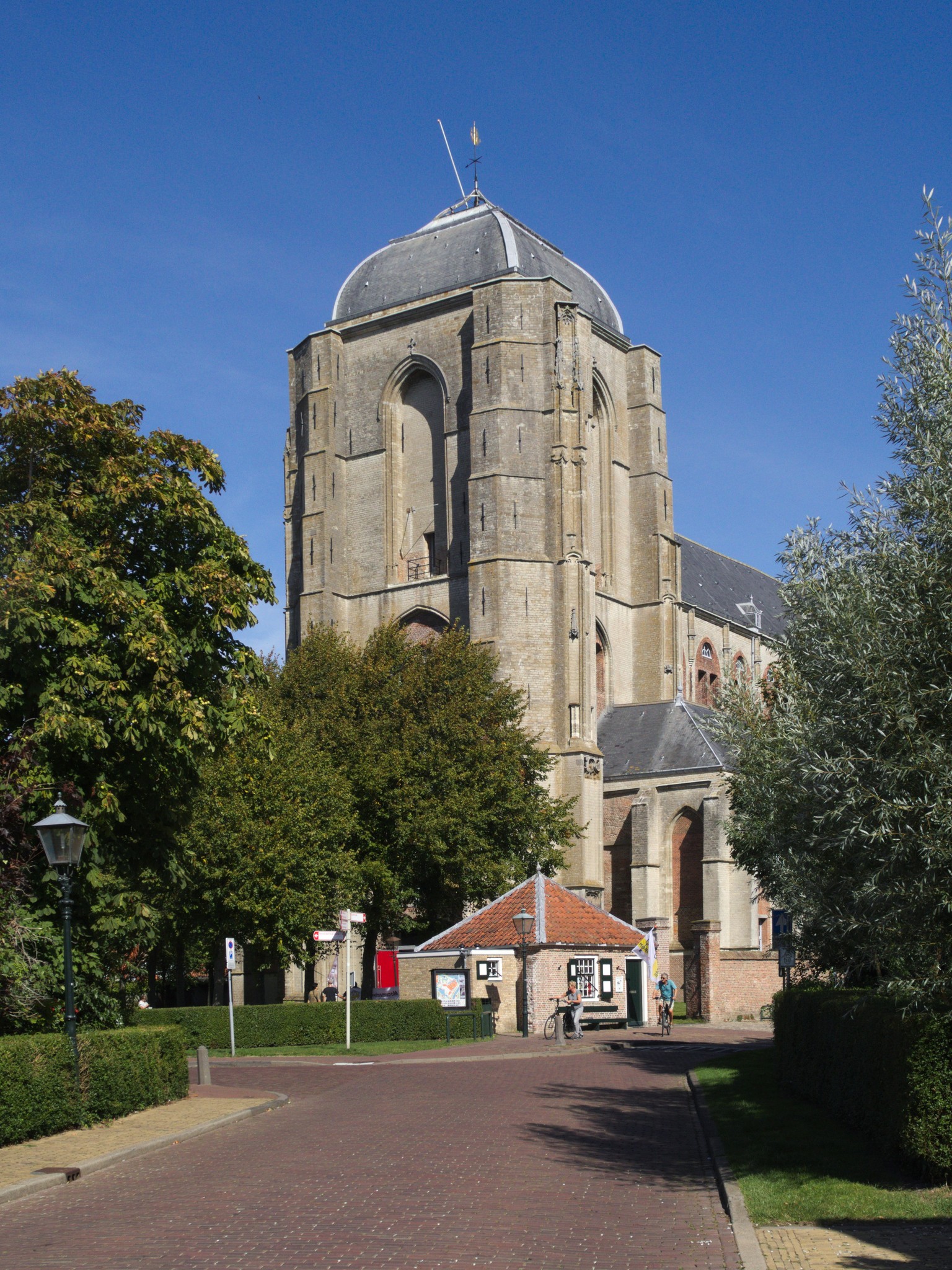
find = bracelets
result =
[571,1000,573,1002]
[674,995,677,997]
[552,997,553,999]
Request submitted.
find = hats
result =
[328,980,333,983]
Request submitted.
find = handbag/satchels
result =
[565,1011,575,1032]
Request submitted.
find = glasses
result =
[661,977,666,979]
[568,985,572,987]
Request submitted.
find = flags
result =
[322,948,338,996]
[632,930,660,984]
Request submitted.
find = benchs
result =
[557,1006,627,1031]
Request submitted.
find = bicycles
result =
[544,998,575,1040]
[652,996,676,1037]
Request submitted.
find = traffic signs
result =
[351,912,366,922]
[339,911,348,930]
[313,930,344,941]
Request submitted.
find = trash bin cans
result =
[481,998,493,1036]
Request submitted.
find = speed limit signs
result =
[780,948,795,968]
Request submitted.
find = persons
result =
[138,994,153,1010]
[652,972,677,1029]
[343,981,366,1001]
[546,980,584,1040]
[322,979,346,1002]
[307,983,321,1003]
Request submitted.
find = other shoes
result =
[658,1021,661,1025]
[669,1024,673,1028]
[578,1035,584,1039]
[573,1036,578,1040]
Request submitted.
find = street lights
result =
[511,905,535,1039]
[385,931,402,986]
[30,791,92,1131]
